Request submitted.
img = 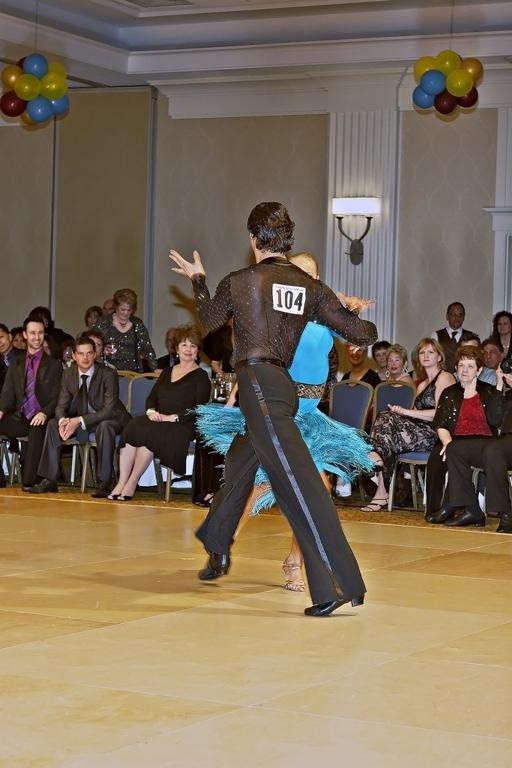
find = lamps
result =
[331,196,382,266]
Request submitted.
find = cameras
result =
[500,357,512,388]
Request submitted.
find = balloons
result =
[407,49,485,115]
[1,54,79,127]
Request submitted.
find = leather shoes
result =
[199,554,230,580]
[305,593,364,616]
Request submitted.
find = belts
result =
[237,358,285,368]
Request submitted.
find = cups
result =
[210,370,236,404]
[107,337,118,360]
[63,346,74,361]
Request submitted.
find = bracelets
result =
[174,414,179,422]
[79,419,82,425]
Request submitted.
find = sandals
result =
[282,557,306,592]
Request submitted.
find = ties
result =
[76,375,89,446]
[22,354,37,420]
[451,332,457,343]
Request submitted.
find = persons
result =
[224,251,377,595]
[322,302,512,533]
[0,288,239,507]
[166,201,378,617]
